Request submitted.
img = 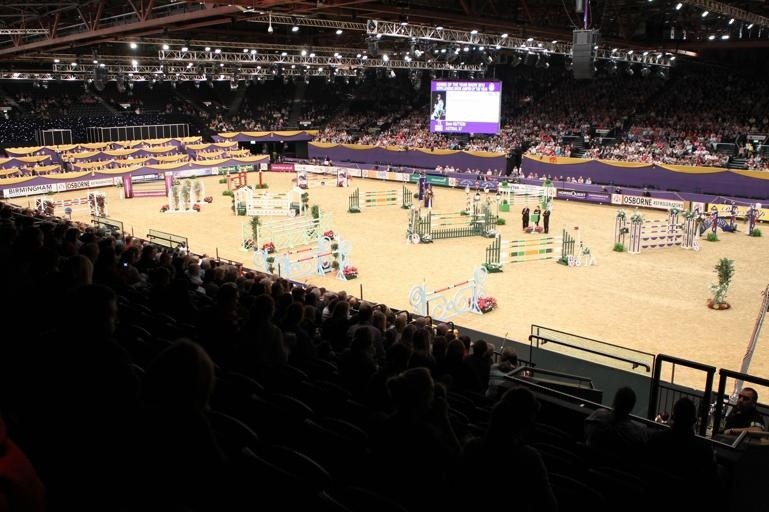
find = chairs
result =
[333,366,642,508]
[106,319,371,507]
